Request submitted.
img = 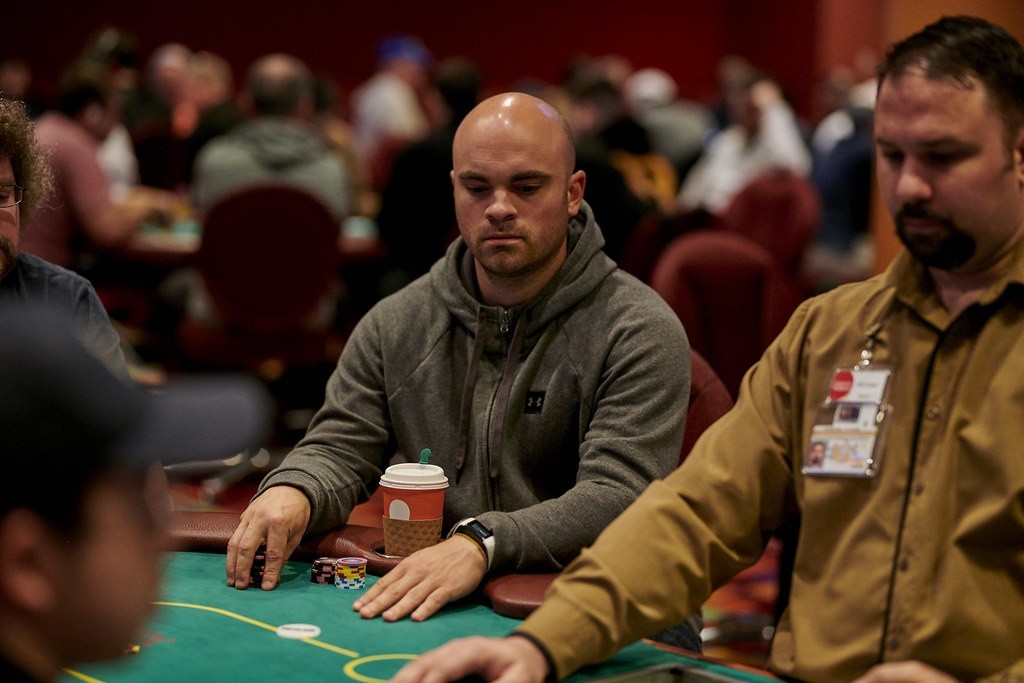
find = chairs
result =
[643,169,820,469]
[198,183,344,406]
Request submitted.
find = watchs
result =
[454,518,494,570]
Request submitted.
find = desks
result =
[118,207,378,263]
[56,511,788,683]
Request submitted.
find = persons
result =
[0,314,168,683]
[392,14,1024,683]
[808,442,826,467]
[0,24,885,484]
[226,91,692,623]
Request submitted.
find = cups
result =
[379,462,450,556]
